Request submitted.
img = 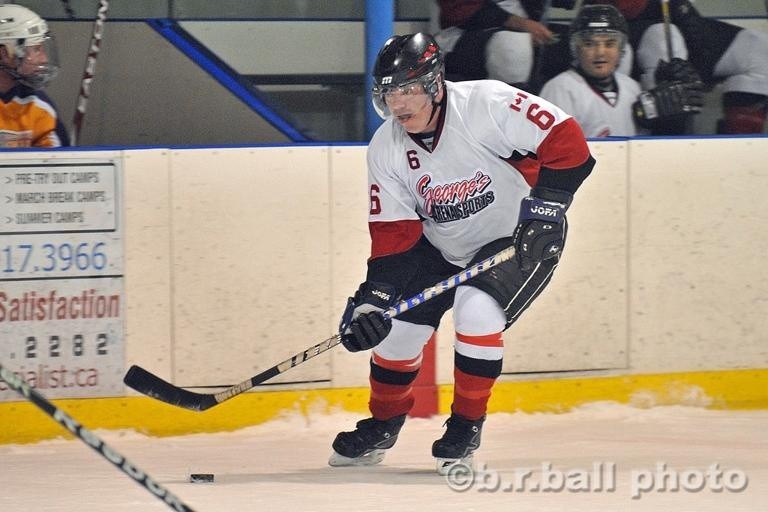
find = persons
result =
[0,3,69,147]
[435,2,768,135]
[328,30,601,462]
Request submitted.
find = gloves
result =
[632,80,705,127]
[513,187,572,278]
[650,57,701,84]
[338,280,400,353]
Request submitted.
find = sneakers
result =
[431,414,486,458]
[332,414,406,458]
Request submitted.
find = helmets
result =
[569,4,626,65]
[0,4,61,92]
[370,32,444,123]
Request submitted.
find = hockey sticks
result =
[124,244,517,410]
